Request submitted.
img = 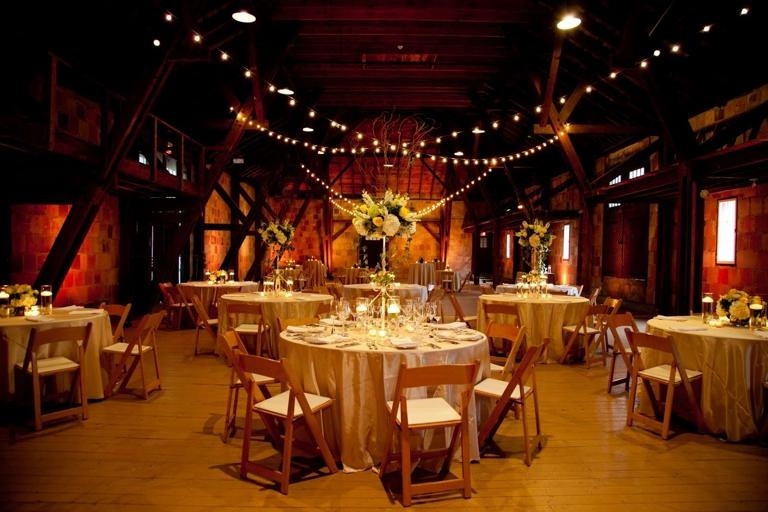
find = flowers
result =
[5,283,38,316]
[209,269,228,285]
[713,288,768,329]
[255,215,296,250]
[349,190,415,244]
[515,219,557,251]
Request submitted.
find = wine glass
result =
[204,268,211,282]
[290,293,484,349]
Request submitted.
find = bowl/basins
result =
[25,310,40,321]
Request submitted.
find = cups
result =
[263,276,293,298]
[229,270,234,282]
[748,296,764,330]
[39,285,53,315]
[0,298,10,320]
[702,292,714,324]
[516,280,547,300]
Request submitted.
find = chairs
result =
[190,295,222,356]
[101,311,162,402]
[476,336,546,467]
[224,347,342,494]
[605,312,645,396]
[377,359,484,506]
[622,328,706,439]
[427,285,446,319]
[13,321,89,432]
[221,327,287,452]
[97,302,132,375]
[482,304,529,376]
[160,281,198,331]
[226,304,274,361]
[560,288,622,364]
[449,292,481,328]
